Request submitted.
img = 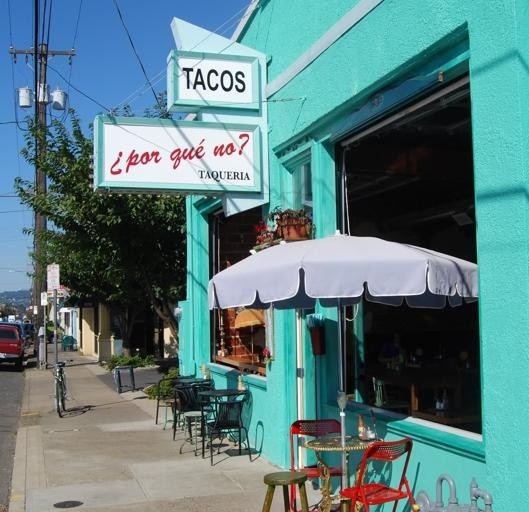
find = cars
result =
[0,320,58,372]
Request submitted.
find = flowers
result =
[253,219,274,244]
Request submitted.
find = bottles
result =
[374,380,383,407]
[200,362,210,380]
[236,375,249,393]
[357,415,376,441]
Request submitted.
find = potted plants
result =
[268,205,314,242]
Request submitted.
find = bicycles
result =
[41,358,74,417]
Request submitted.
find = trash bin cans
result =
[62,335,73,351]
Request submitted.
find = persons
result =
[378,331,407,368]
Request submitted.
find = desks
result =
[412,407,481,425]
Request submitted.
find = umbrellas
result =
[206,230,479,447]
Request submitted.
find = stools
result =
[262,471,309,512]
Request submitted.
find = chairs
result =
[290,418,417,512]
[372,375,461,415]
[156,374,252,466]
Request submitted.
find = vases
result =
[261,237,270,244]
